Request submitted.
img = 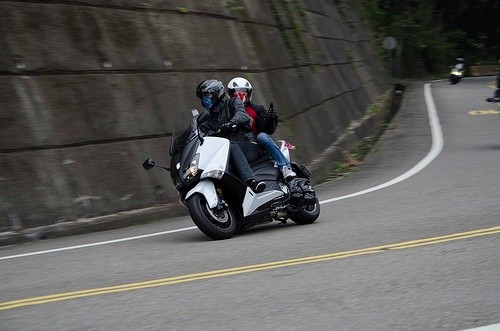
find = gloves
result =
[268,102,275,119]
[220,122,232,135]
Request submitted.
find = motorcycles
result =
[449,68,464,84]
[142,109,321,240]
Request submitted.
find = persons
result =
[454,58,466,72]
[196,78,268,193]
[225,76,297,183]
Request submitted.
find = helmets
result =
[226,77,253,102]
[196,79,225,103]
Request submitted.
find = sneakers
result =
[250,179,267,193]
[280,166,297,181]
